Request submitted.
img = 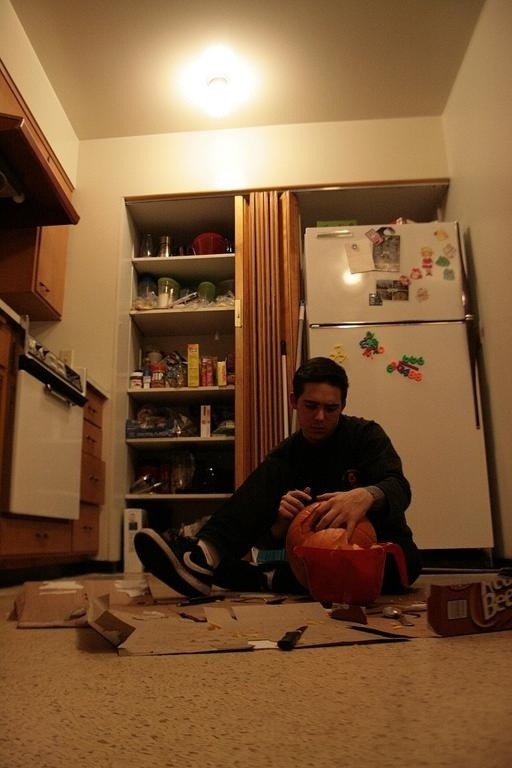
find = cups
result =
[293,541,415,607]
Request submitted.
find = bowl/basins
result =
[191,233,226,254]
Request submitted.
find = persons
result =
[134,357,421,602]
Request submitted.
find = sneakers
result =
[212,556,291,596]
[130,520,216,602]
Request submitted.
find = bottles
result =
[140,232,186,256]
[143,358,152,376]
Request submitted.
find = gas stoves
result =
[22,331,89,409]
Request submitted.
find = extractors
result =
[0,112,81,228]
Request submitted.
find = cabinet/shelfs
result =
[0,303,21,514]
[123,194,240,502]
[0,112,72,321]
[0,513,72,569]
[72,384,106,560]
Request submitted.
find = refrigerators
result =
[298,219,495,567]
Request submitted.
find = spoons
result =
[383,606,414,627]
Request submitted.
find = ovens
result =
[6,367,86,523]
